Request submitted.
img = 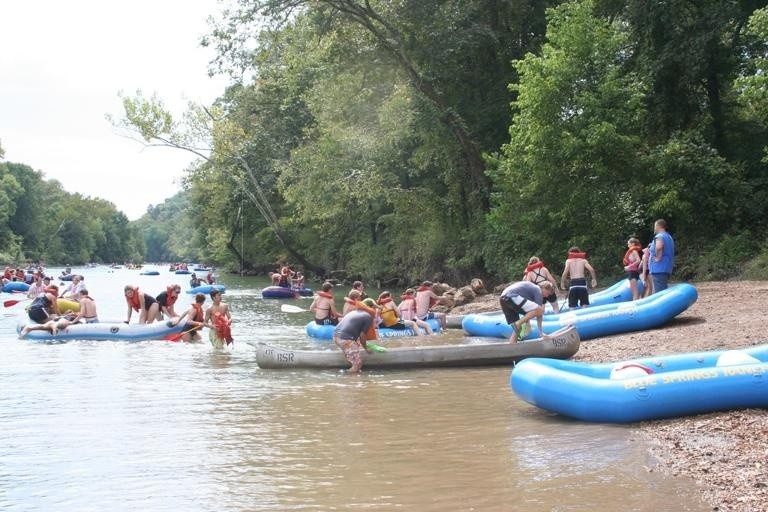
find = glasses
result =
[175,291,180,294]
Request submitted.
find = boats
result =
[185,284,226,298]
[59,272,84,283]
[246,322,581,371]
[260,286,314,300]
[88,260,215,276]
[17,314,190,343]
[460,271,698,339]
[431,310,506,328]
[0,280,31,294]
[506,342,767,425]
[302,316,442,342]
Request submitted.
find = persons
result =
[560,247,597,311]
[522,257,560,314]
[499,280,555,343]
[649,219,674,293]
[624,238,640,300]
[639,243,652,298]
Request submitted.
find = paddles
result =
[162,326,199,342]
[280,304,310,314]
[4,298,31,307]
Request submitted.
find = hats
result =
[363,298,383,310]
